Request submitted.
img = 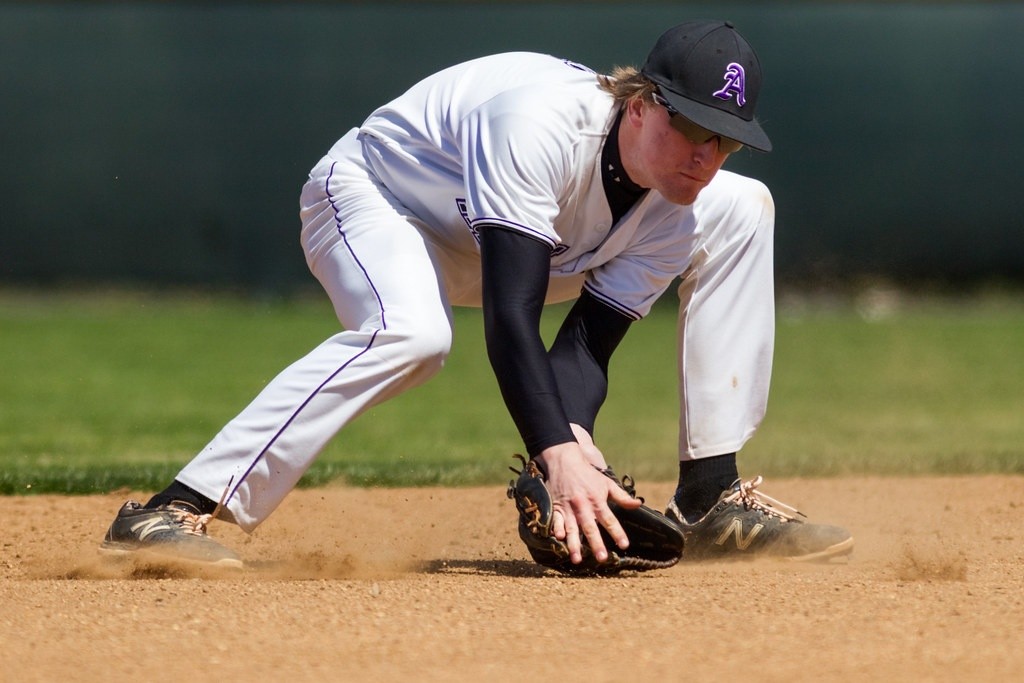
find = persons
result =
[97,19,853,567]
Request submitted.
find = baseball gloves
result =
[506,453,688,571]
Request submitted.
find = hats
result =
[641,21,772,154]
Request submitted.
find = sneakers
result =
[96,475,248,573]
[663,476,853,564]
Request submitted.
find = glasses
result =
[650,92,744,154]
